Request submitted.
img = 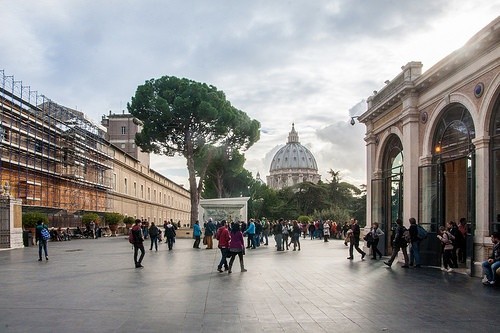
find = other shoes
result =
[362,253,366,258]
[218,269,223,272]
[344,242,348,246]
[241,269,247,272]
[483,281,495,286]
[46,256,49,260]
[371,258,376,260]
[441,268,454,273]
[347,256,353,259]
[228,271,232,273]
[135,262,143,268]
[225,267,229,270]
[401,265,409,268]
[379,254,382,258]
[384,261,392,267]
[38,258,42,261]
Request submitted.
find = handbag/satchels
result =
[203,237,207,244]
[128,230,135,244]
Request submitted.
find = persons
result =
[29,227,71,241]
[384,219,409,268]
[347,218,366,259]
[407,218,421,268]
[370,221,384,260]
[228,223,247,273]
[132,219,145,268]
[35,219,49,261]
[193,220,202,249]
[390,223,397,255]
[74,226,82,238]
[248,217,352,251]
[481,231,500,284]
[148,222,159,251]
[437,217,468,272]
[215,220,231,272]
[243,218,256,249]
[203,218,246,248]
[142,219,162,242]
[164,219,180,250]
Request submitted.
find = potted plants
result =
[21,210,50,236]
[81,212,101,230]
[102,212,125,231]
[123,217,135,229]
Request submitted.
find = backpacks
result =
[40,224,51,240]
[402,230,412,242]
[416,224,428,239]
